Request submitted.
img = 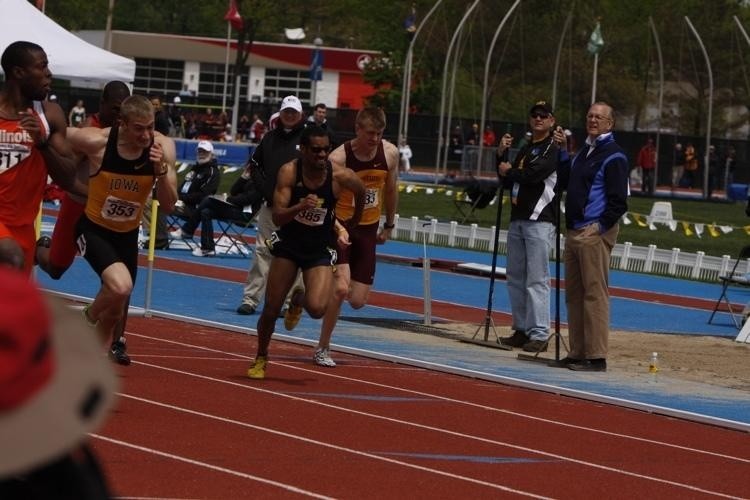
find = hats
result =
[529,101,553,112]
[195,140,214,152]
[280,95,302,113]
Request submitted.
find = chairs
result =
[212,199,267,259]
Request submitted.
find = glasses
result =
[531,112,548,119]
[309,144,330,153]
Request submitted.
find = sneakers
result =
[568,358,607,371]
[523,339,548,352]
[145,239,168,250]
[80,305,99,329]
[108,337,131,367]
[191,247,215,257]
[32,236,51,265]
[237,304,255,314]
[284,285,304,331]
[170,228,193,240]
[312,346,336,367]
[497,329,529,347]
[548,356,581,367]
[248,354,268,379]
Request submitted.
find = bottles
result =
[647,351,659,385]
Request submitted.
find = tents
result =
[2,0,135,90]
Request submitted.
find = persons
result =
[671,143,685,186]
[36,79,132,364]
[67,96,178,347]
[248,126,366,378]
[238,115,248,140]
[171,164,262,257]
[69,100,86,126]
[0,41,78,274]
[145,139,221,250]
[314,105,399,367]
[492,99,566,354]
[637,138,655,193]
[169,104,185,138]
[483,124,496,146]
[216,112,228,127]
[686,144,699,186]
[467,123,479,147]
[237,96,305,314]
[702,146,718,188]
[548,102,629,372]
[307,103,328,136]
[450,126,465,155]
[150,98,169,137]
[398,139,413,172]
[201,108,215,124]
[249,113,263,142]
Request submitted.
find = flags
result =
[220,0,250,36]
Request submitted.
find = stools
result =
[165,213,200,252]
